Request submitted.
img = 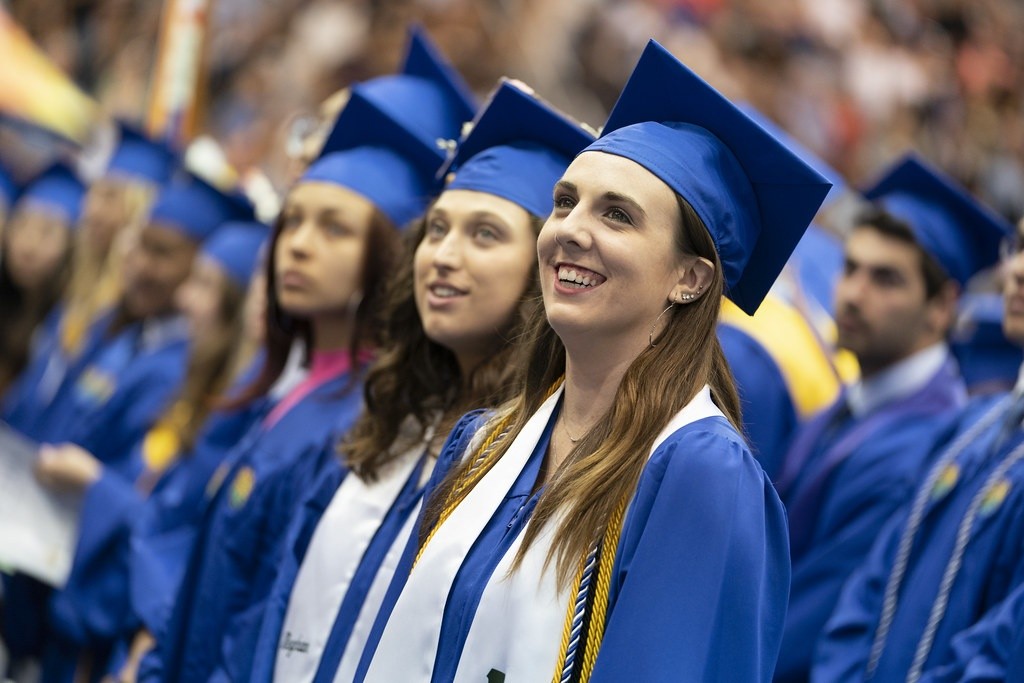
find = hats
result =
[859,153,1017,295]
[16,163,88,219]
[200,218,271,287]
[301,92,454,230]
[437,81,597,221]
[0,168,18,205]
[153,166,255,241]
[575,38,834,316]
[348,33,479,161]
[108,119,181,188]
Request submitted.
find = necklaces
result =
[563,414,582,442]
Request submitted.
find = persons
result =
[712,97,1024,683]
[0,27,833,683]
